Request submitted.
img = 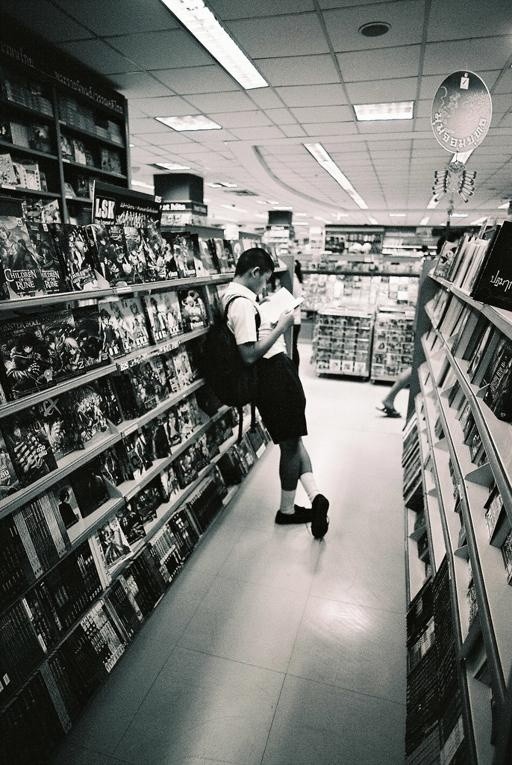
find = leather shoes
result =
[275,494,328,537]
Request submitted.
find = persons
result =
[290,257,304,377]
[223,246,337,539]
[375,363,412,418]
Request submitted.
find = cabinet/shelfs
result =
[398,222,511,765]
[1,46,132,227]
[1,230,296,764]
[301,228,414,382]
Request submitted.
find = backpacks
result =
[198,296,260,407]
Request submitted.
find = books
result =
[309,219,400,384]
[255,287,304,327]
[401,217,510,765]
[1,35,271,764]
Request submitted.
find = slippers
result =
[377,405,400,417]
